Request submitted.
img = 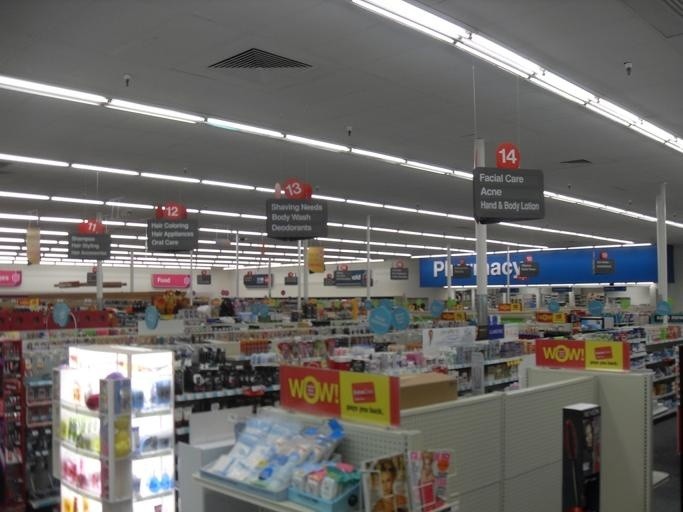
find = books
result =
[407,448,450,512]
[361,451,407,512]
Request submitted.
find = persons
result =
[581,420,595,477]
[416,452,447,511]
[371,461,405,512]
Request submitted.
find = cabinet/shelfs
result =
[0,297,683,512]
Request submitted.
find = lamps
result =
[0,0,683,268]
